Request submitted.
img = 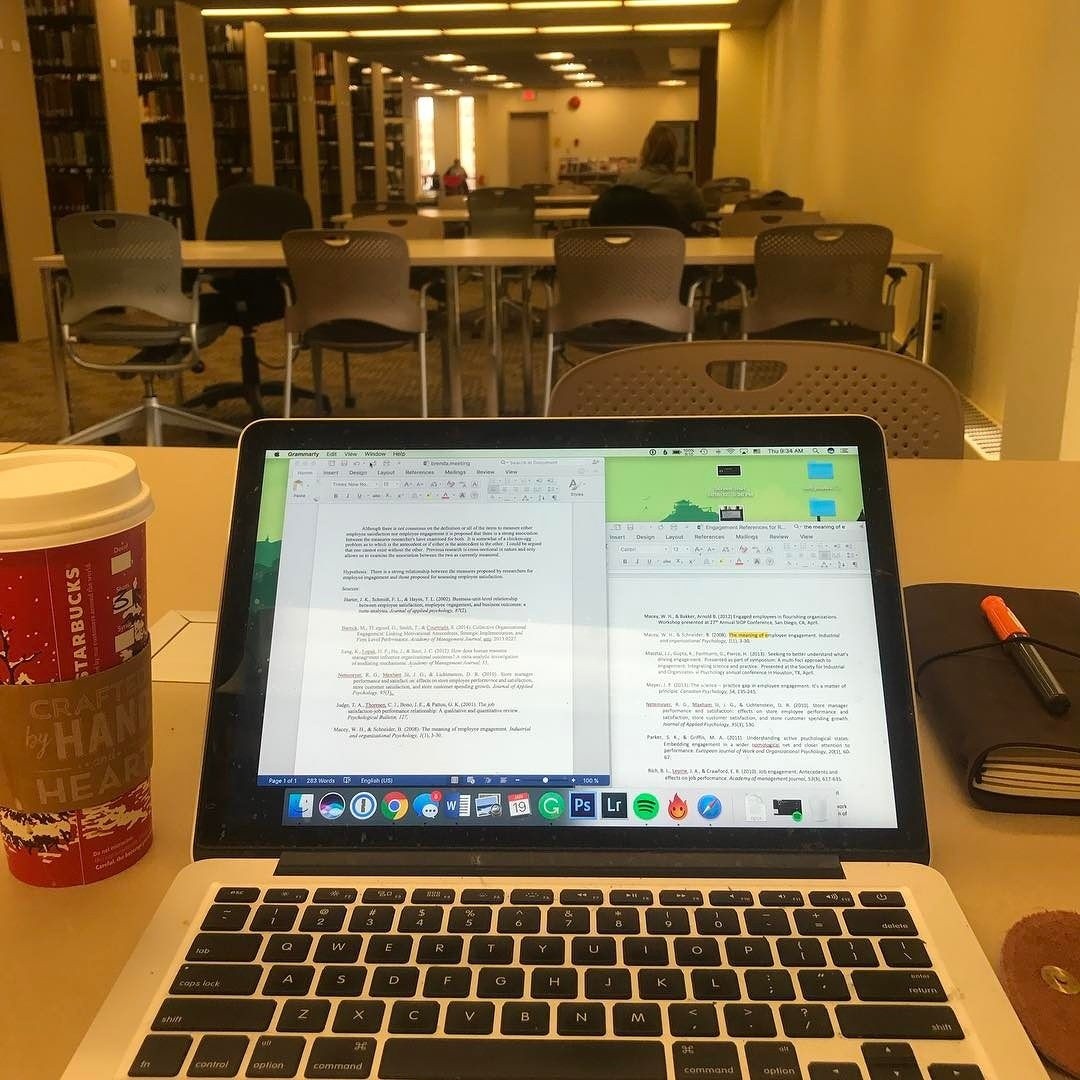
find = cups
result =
[0,447,154,888]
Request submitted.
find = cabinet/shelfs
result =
[30,16,404,310]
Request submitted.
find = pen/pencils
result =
[980,596,1069,710]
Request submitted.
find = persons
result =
[432,159,470,196]
[616,122,709,237]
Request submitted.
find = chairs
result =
[50,176,964,456]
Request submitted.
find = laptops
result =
[59,413,1054,1080]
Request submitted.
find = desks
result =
[0,439,1080,1080]
[329,203,735,334]
[33,235,941,435]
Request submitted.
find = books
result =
[558,155,642,175]
[25,0,404,256]
[902,583,1080,816]
